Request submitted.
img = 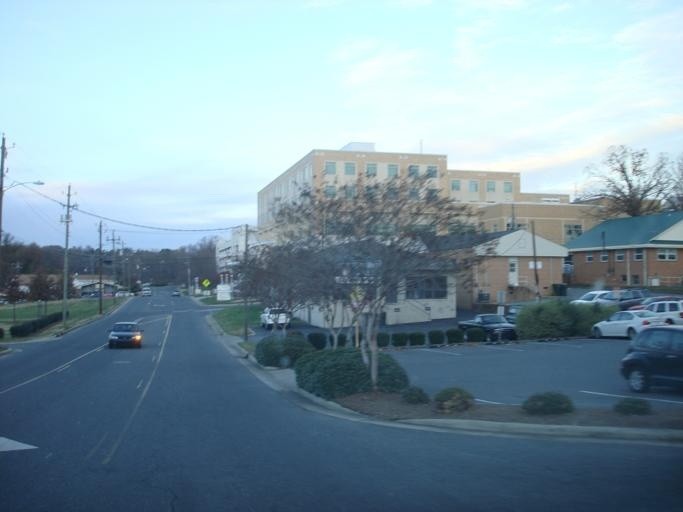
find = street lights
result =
[0,180,46,242]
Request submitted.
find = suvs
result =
[260,306,295,329]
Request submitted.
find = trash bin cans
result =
[360,311,386,326]
[552,282,568,296]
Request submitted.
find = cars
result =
[171,290,180,296]
[84,291,135,297]
[455,286,683,343]
[140,288,151,296]
[107,321,144,349]
[621,321,682,395]
